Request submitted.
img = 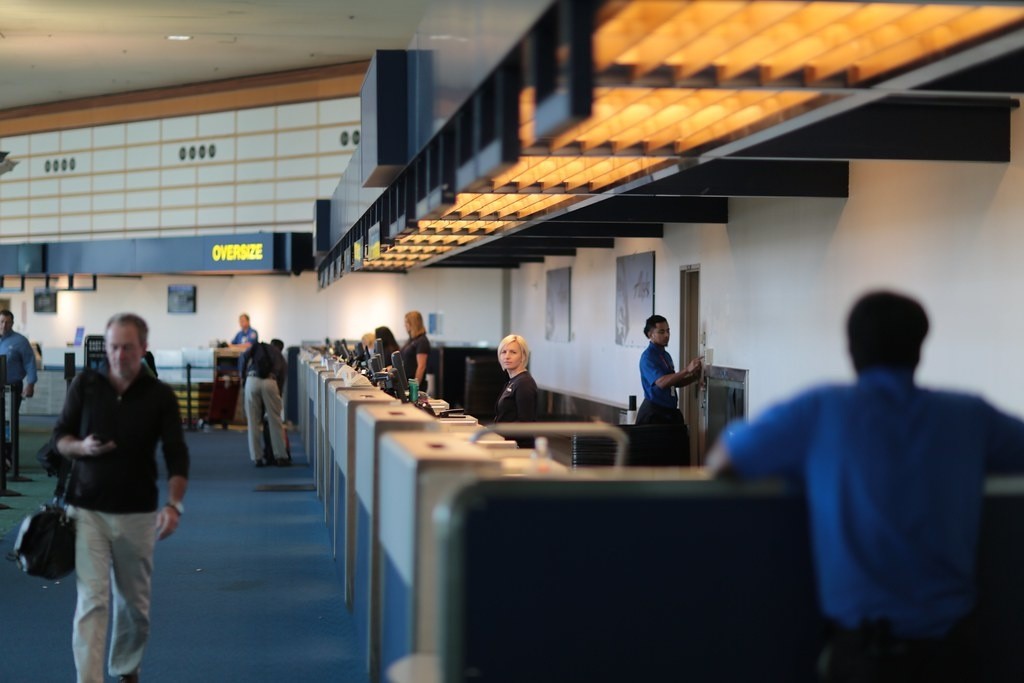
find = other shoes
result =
[249,458,263,466]
[277,456,289,464]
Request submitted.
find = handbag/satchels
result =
[13,504,77,581]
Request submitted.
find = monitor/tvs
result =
[326,338,408,404]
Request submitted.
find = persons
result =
[231,314,258,378]
[362,333,376,354]
[708,293,1024,683]
[238,339,290,468]
[386,311,430,391]
[494,334,542,449]
[634,315,706,424]
[0,309,38,470]
[50,314,191,683]
[375,326,399,367]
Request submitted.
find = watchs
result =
[166,501,184,514]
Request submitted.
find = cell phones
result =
[94,435,111,446]
